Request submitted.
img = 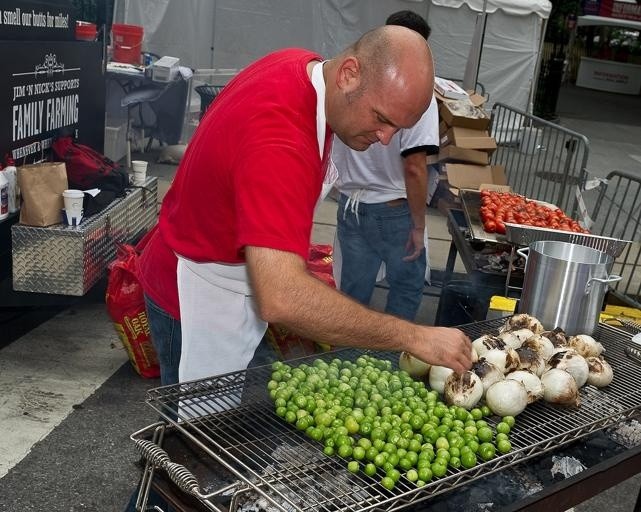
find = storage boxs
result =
[426,77,510,215]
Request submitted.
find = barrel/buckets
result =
[75,20,97,41]
[112,24,145,66]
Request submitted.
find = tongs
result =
[134,312,641,512]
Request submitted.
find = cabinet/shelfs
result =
[11,174,158,297]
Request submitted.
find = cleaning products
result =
[0,158,22,220]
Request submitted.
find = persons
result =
[549,41,565,79]
[139,25,471,428]
[330,10,439,322]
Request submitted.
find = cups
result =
[62,188,86,226]
[131,160,149,186]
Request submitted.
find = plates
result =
[503,222,631,259]
[457,187,567,250]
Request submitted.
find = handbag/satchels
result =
[54,133,129,197]
[18,142,68,227]
